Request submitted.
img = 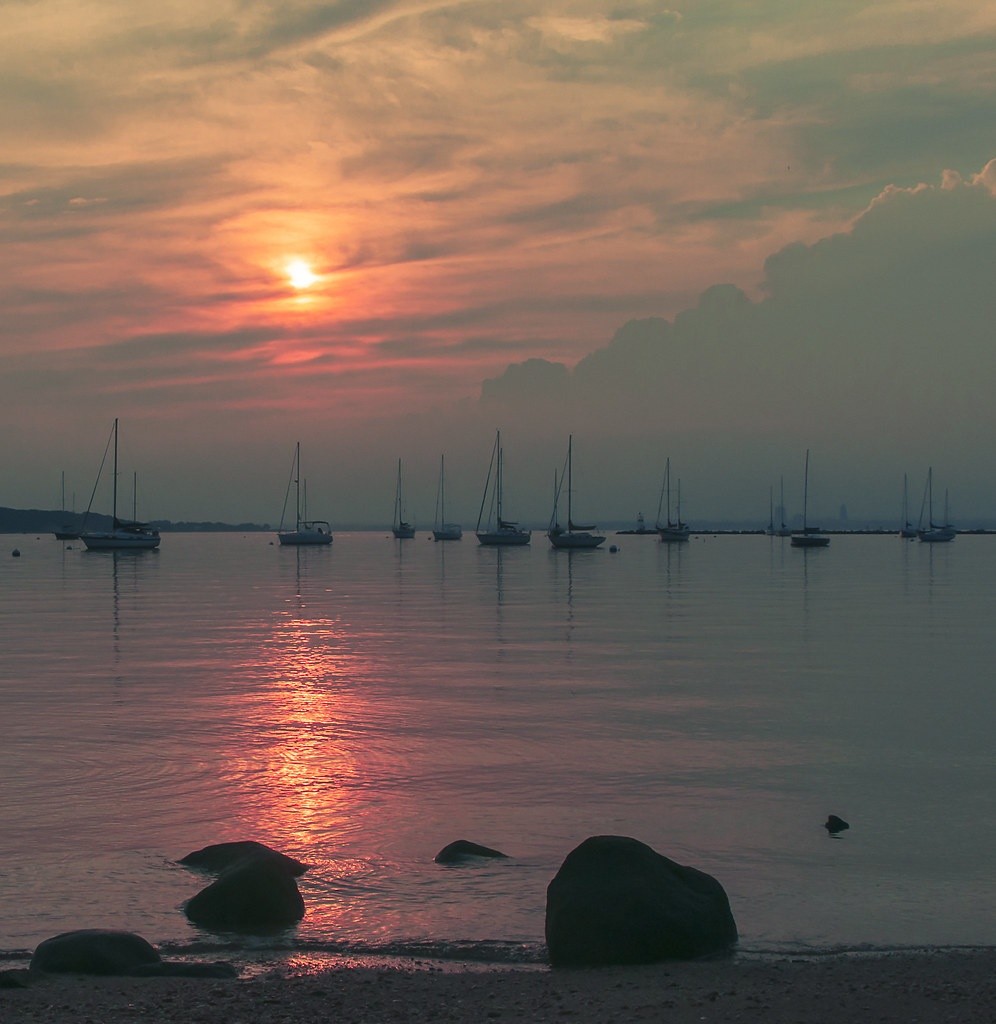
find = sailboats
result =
[915,466,955,542]
[53,469,82,539]
[276,440,333,544]
[430,452,464,541]
[652,457,691,542]
[475,430,534,545]
[79,417,162,549]
[897,470,920,536]
[766,485,776,536]
[389,457,418,538]
[776,471,789,537]
[788,448,832,547]
[547,433,604,548]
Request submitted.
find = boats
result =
[570,522,598,532]
